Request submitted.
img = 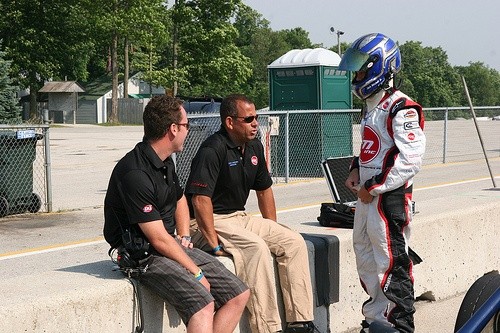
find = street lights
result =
[330,27,344,56]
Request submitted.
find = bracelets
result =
[182,236,191,242]
[212,246,222,252]
[194,270,203,280]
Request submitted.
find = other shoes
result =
[286,321,317,333]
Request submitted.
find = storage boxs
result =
[322,154,417,213]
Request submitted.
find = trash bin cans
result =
[174,102,222,189]
[0,130,43,218]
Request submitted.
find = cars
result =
[182,102,221,132]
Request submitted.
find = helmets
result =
[351,33,402,100]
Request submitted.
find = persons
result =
[103,96,250,333]
[339,33,426,333]
[185,94,314,333]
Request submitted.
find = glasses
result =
[168,122,190,129]
[229,115,258,123]
